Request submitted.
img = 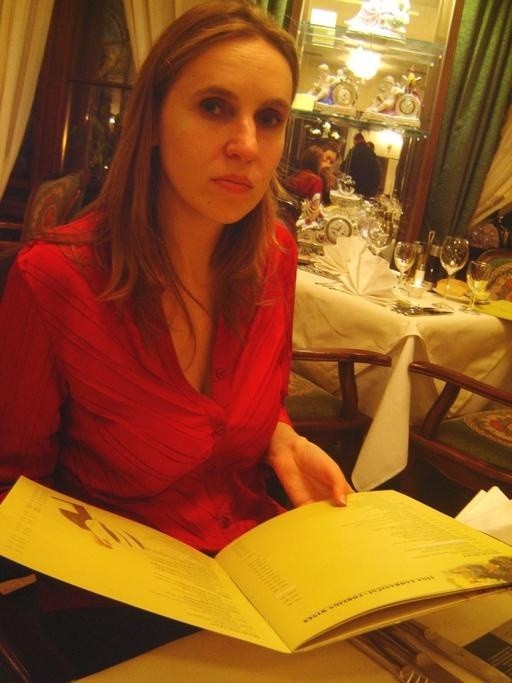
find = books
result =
[0,472,512,655]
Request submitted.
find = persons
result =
[284,134,384,208]
[0,1,355,683]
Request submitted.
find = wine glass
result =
[391,241,417,290]
[433,237,491,314]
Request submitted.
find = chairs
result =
[1,168,90,274]
[286,345,510,500]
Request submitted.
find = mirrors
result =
[289,2,462,243]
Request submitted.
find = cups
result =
[367,219,393,255]
